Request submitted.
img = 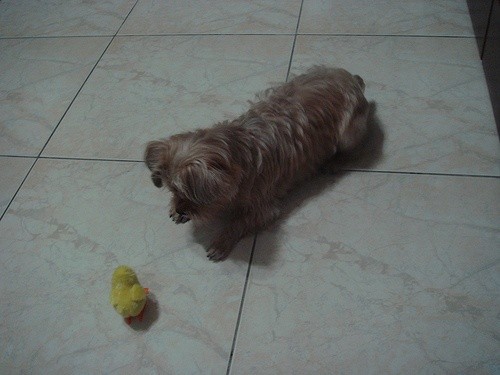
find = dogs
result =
[144,64,369,262]
